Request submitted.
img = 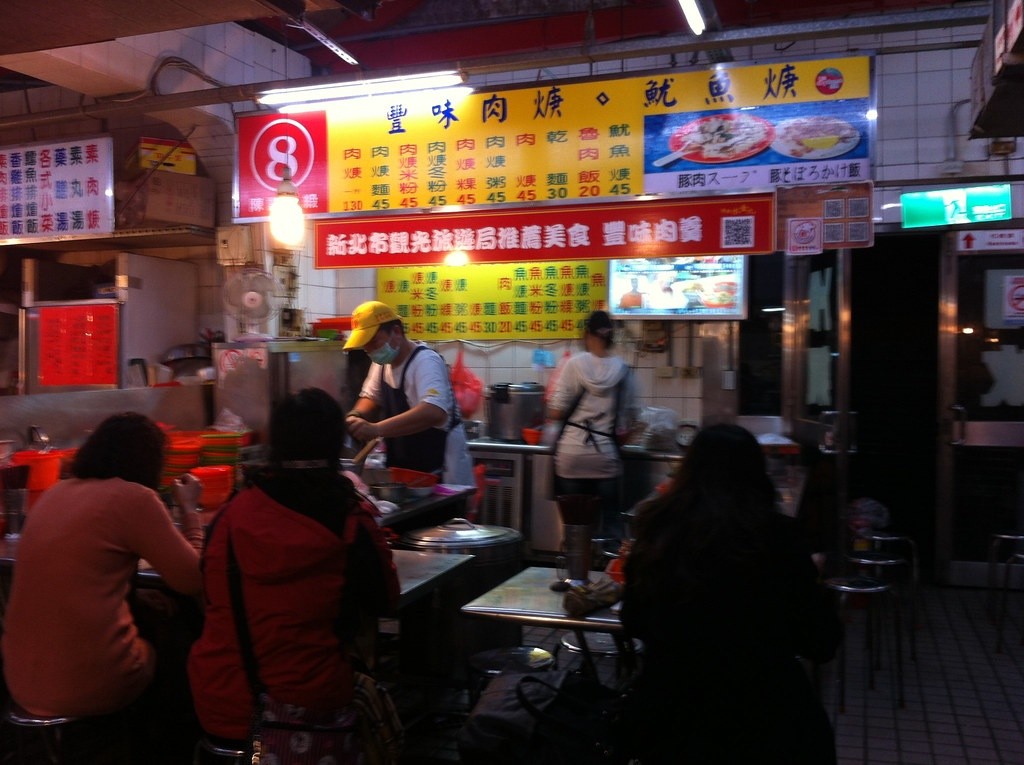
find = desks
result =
[0,533,477,677]
[464,567,668,712]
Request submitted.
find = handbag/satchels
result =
[247,673,404,765]
[455,666,641,765]
[449,351,483,413]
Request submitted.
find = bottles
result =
[128,358,147,388]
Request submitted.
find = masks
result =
[368,334,402,366]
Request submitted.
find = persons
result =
[543,311,640,569]
[0,411,205,765]
[187,387,403,765]
[342,301,477,529]
[619,423,845,765]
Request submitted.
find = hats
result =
[342,301,401,349]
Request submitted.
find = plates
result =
[670,112,862,164]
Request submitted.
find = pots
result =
[400,518,523,601]
[340,458,364,480]
[481,381,546,439]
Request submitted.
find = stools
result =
[191,734,247,765]
[0,701,84,765]
[550,631,645,683]
[833,527,1024,712]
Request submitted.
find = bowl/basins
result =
[521,427,543,445]
[388,467,439,488]
[155,415,255,510]
[369,482,406,504]
[307,316,353,340]
[11,447,80,515]
[408,486,434,497]
[161,344,210,363]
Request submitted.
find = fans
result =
[220,267,289,347]
